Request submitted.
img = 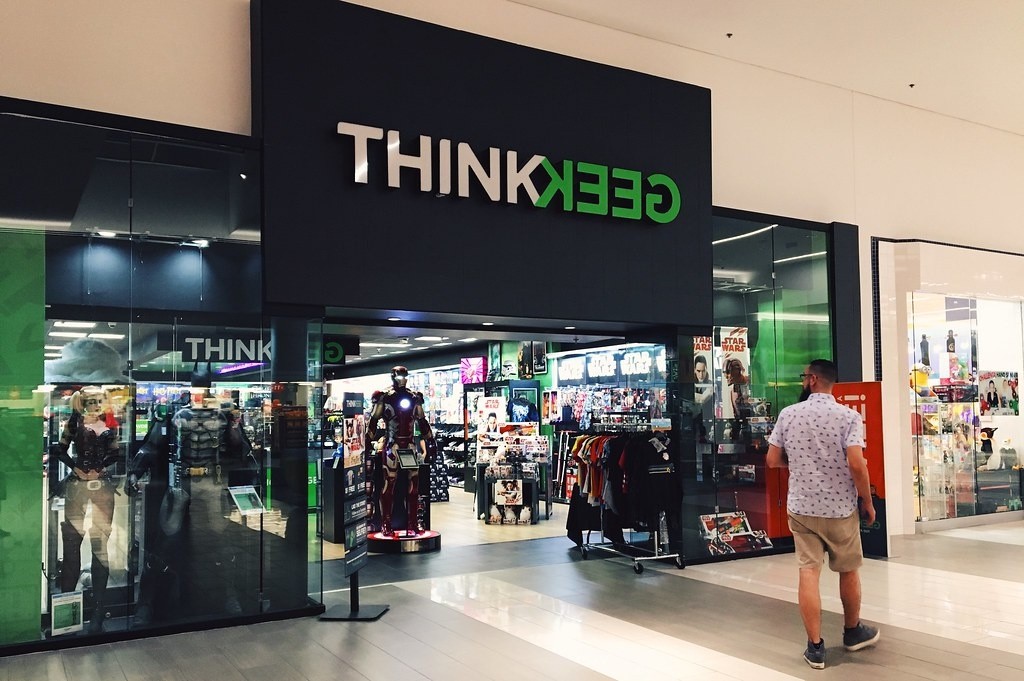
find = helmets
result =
[723,358,743,372]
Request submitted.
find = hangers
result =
[583,421,667,441]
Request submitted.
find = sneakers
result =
[843,621,881,652]
[803,637,826,669]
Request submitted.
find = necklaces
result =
[85,417,98,425]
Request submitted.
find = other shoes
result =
[88,608,104,633]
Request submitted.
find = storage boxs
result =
[719,415,778,483]
[698,510,774,555]
[429,451,450,503]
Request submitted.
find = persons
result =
[365,366,437,536]
[486,412,497,432]
[497,480,518,503]
[987,380,999,407]
[355,417,363,436]
[998,437,1017,469]
[44,386,121,638]
[722,358,749,417]
[507,394,537,422]
[123,360,259,627]
[765,359,880,669]
[694,355,712,394]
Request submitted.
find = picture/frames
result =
[531,341,548,375]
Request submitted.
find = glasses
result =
[800,373,819,382]
